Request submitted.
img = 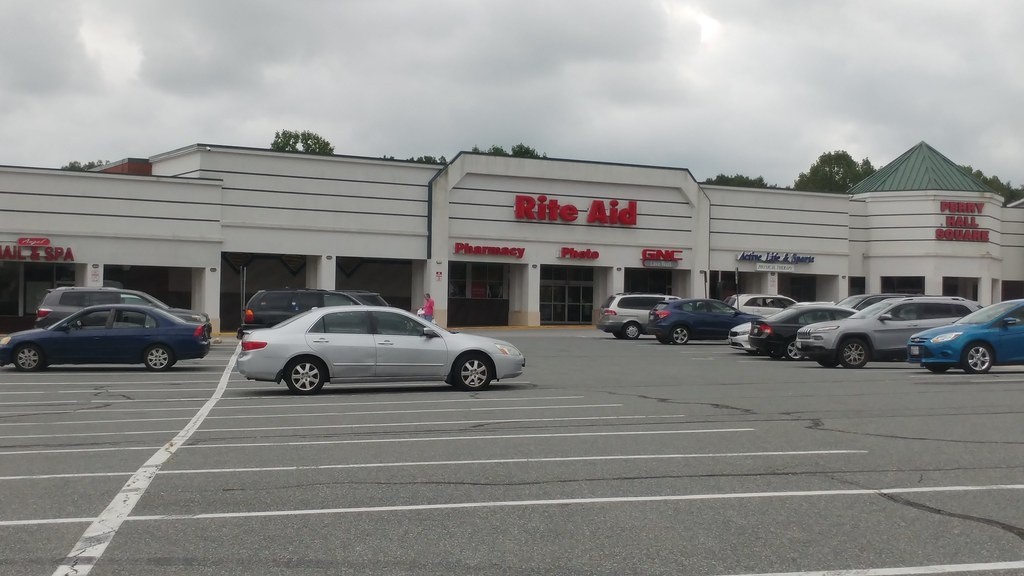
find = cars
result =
[748,302,860,362]
[906,299,1024,373]
[237,304,524,393]
[728,319,769,356]
[0,304,210,372]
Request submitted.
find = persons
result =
[421,293,434,322]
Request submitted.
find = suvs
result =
[234,287,392,343]
[795,295,979,370]
[646,296,765,345]
[834,292,923,313]
[723,292,798,319]
[33,285,213,339]
[595,292,683,341]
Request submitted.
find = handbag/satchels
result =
[416,308,425,319]
